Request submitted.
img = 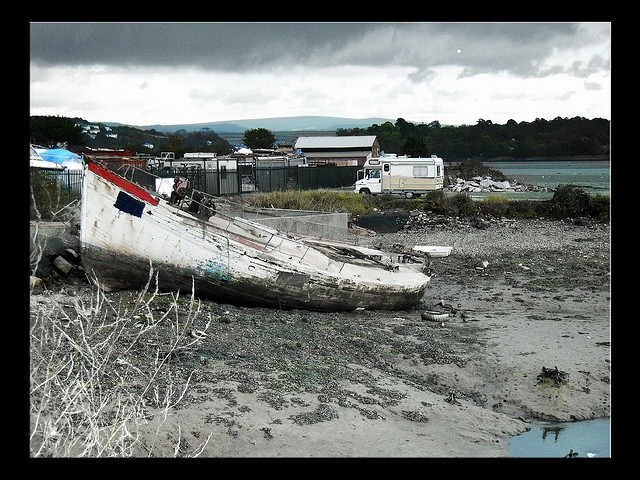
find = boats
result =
[78,158,430,309]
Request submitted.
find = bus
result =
[353,151,445,198]
